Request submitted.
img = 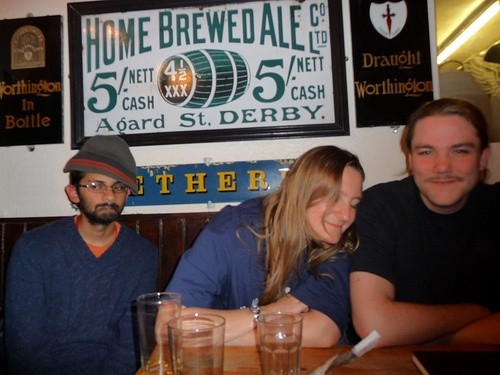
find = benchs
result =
[0,214,220,375]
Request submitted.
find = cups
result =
[167,313,226,375]
[136,292,182,375]
[253,313,303,375]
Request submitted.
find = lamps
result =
[435,0,500,68]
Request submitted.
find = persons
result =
[5,134,159,375]
[152,145,364,348]
[348,96,500,351]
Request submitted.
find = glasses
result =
[74,181,128,193]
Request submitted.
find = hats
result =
[63,135,138,195]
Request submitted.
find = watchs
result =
[249,303,260,328]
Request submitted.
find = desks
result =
[135,341,500,375]
